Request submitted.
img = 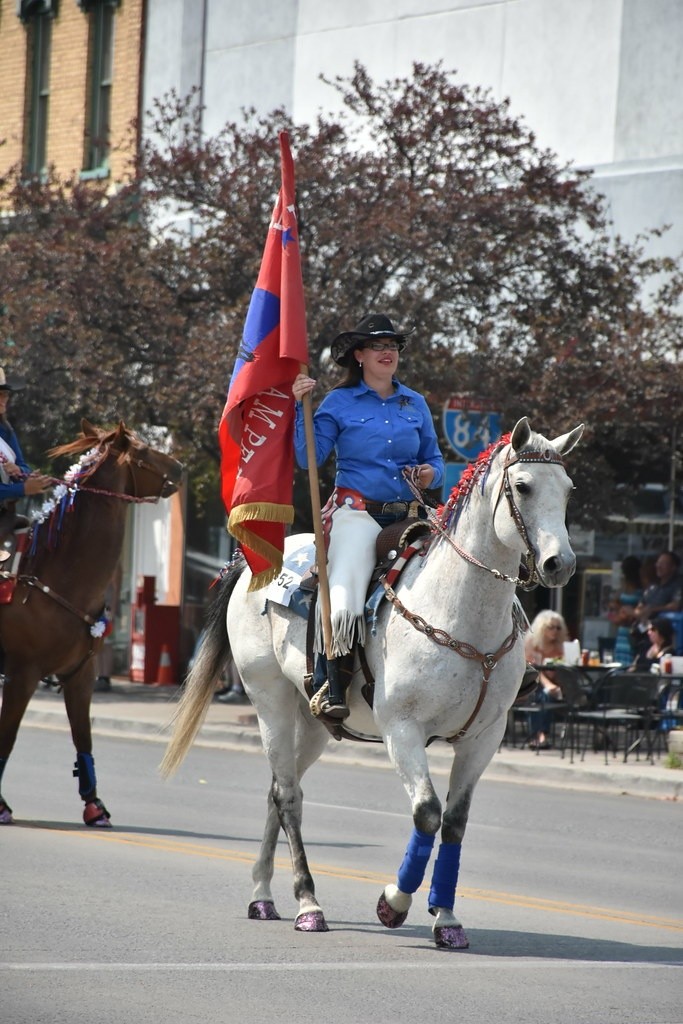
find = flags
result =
[218,134,307,592]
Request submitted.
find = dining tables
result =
[529,661,630,753]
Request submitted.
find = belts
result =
[367,500,409,514]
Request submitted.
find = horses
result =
[151,415,585,951]
[2,415,187,828]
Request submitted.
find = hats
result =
[330,313,416,368]
[0,367,27,392]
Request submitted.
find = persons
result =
[0,368,52,536]
[627,617,678,674]
[292,314,443,718]
[523,609,569,749]
[213,658,243,704]
[608,552,683,672]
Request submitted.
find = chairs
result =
[499,665,683,766]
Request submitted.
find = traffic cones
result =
[151,644,175,687]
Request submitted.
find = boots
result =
[313,650,352,718]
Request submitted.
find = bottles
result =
[590,651,599,666]
[582,650,589,665]
[665,654,672,674]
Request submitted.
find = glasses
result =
[364,342,400,351]
[548,623,562,630]
